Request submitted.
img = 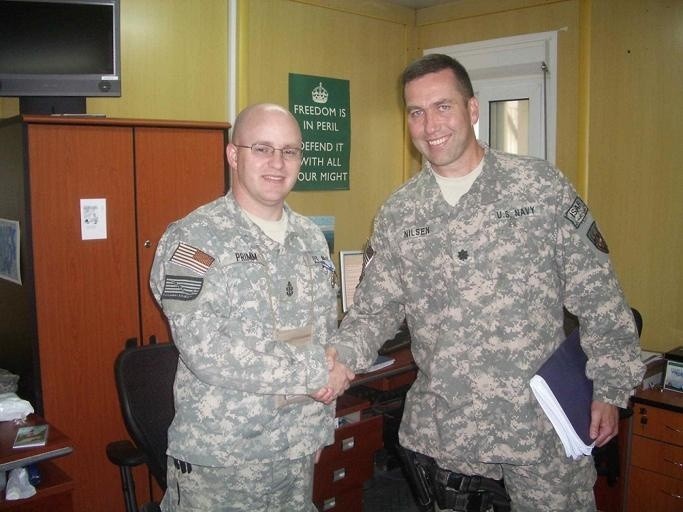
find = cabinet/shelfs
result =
[1,116,231,512]
[0,407,76,512]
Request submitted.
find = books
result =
[12,423,47,449]
[529,326,597,461]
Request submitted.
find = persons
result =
[148,102,356,511]
[312,54,648,511]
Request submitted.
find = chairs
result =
[105,341,182,511]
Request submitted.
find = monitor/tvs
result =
[0,0,121,118]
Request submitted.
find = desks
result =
[310,345,424,511]
[620,389,682,512]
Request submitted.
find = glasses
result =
[235,144,306,161]
